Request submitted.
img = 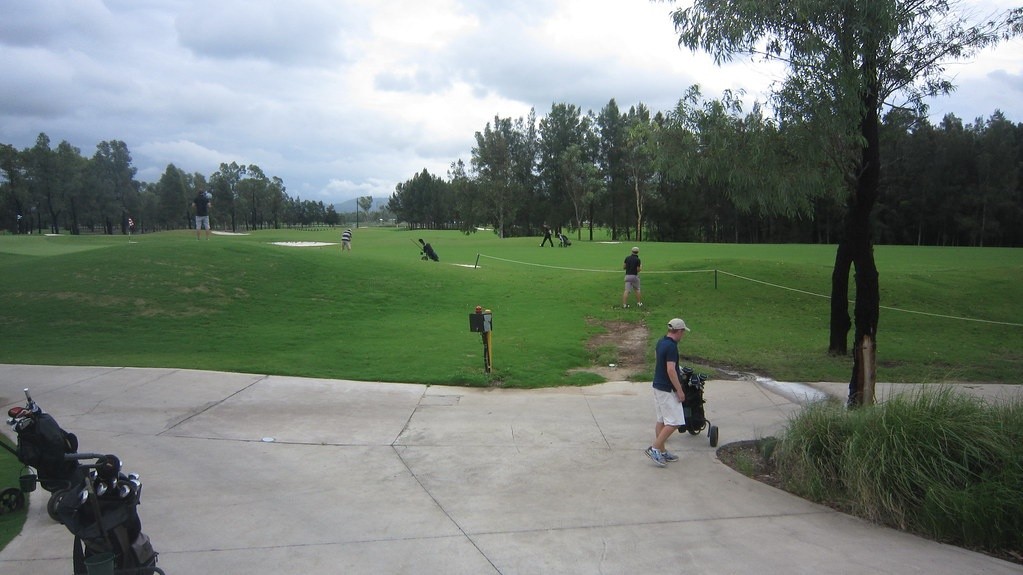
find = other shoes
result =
[637,303,643,307]
[623,304,631,309]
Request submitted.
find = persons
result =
[192,188,212,240]
[643,318,691,467]
[622,247,643,307]
[341,229,352,253]
[540,223,554,247]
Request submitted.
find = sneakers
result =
[644,446,679,466]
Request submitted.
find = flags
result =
[129,218,134,227]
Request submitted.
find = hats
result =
[668,318,690,332]
[632,246,639,252]
